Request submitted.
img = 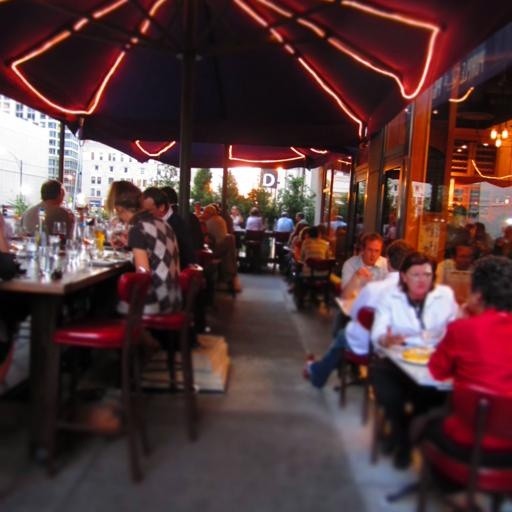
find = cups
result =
[94,226,105,256]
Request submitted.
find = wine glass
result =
[53,221,81,270]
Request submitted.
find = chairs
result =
[334,306,377,424]
[385,383,511,511]
[235,229,291,273]
[51,243,213,481]
[297,256,337,308]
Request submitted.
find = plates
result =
[14,250,124,266]
[396,334,438,365]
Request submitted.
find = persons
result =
[274,199,512,512]
[1,176,273,439]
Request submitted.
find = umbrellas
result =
[0,0,459,227]
[68,127,360,225]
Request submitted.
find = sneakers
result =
[303,352,316,381]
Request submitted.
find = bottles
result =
[32,208,52,278]
